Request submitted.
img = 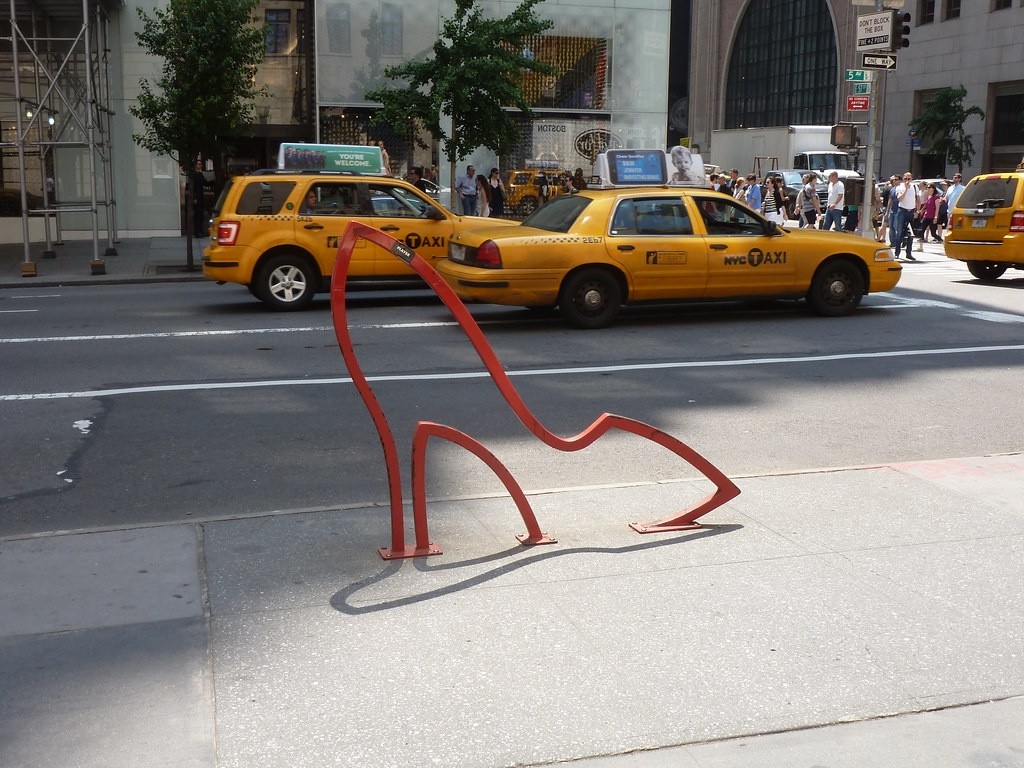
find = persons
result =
[454,165,506,219]
[285,146,325,169]
[188,160,220,239]
[299,190,321,215]
[794,171,966,261]
[670,146,705,185]
[564,168,588,193]
[702,168,789,230]
[409,166,426,194]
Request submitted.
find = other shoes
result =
[906,255,916,260]
[895,255,900,259]
[875,239,944,248]
[913,248,923,252]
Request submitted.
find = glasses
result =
[953,177,958,179]
[892,179,898,181]
[565,179,570,182]
[904,178,911,180]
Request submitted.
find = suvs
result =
[943,153,1024,281]
[199,141,526,311]
[502,153,570,216]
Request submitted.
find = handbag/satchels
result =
[478,202,490,217]
[500,188,507,201]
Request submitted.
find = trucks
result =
[710,124,863,183]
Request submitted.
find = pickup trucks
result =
[759,170,828,220]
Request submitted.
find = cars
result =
[371,176,459,211]
[915,179,963,194]
[435,149,903,314]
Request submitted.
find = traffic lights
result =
[883,6,911,52]
[830,124,855,146]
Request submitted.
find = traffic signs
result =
[844,69,874,95]
[862,53,898,71]
[846,95,869,111]
